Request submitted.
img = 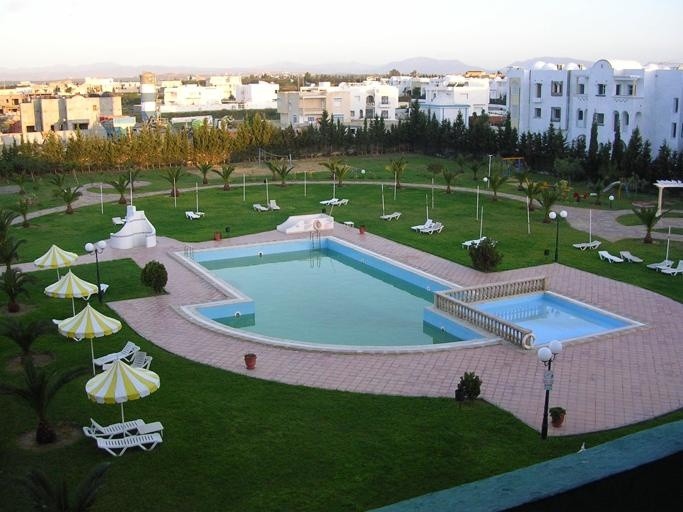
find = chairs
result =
[111,196,349,226]
[572,240,683,277]
[380,211,497,248]
[81,282,164,458]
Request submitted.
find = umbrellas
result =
[85,358,160,436]
[56,301,121,376]
[34,244,78,280]
[42,268,98,318]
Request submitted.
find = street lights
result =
[85,240,106,302]
[549,209,568,261]
[538,340,562,440]
